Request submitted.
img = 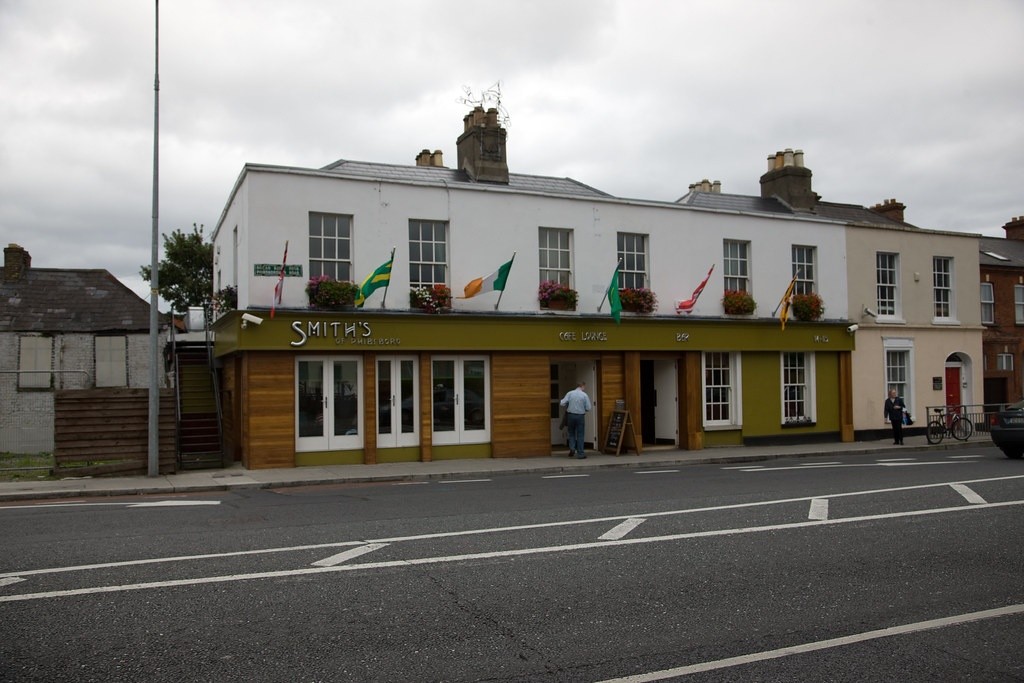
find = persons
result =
[560,381,591,459]
[884,389,906,445]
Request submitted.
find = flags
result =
[607,258,622,324]
[780,269,801,331]
[271,241,289,319]
[675,264,715,314]
[455,252,516,299]
[354,247,394,308]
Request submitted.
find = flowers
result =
[537,280,579,310]
[410,284,452,315]
[304,274,358,311]
[792,292,825,322]
[720,289,757,316]
[619,288,659,320]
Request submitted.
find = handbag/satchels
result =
[902,407,913,425]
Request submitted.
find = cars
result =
[990,400,1024,459]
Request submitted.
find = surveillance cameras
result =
[242,313,263,325]
[847,325,859,332]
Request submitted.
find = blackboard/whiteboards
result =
[602,410,639,450]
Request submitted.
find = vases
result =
[623,303,651,312]
[548,297,575,311]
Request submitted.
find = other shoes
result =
[893,441,904,445]
[569,450,575,457]
[579,456,587,459]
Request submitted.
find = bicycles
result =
[925,406,973,445]
